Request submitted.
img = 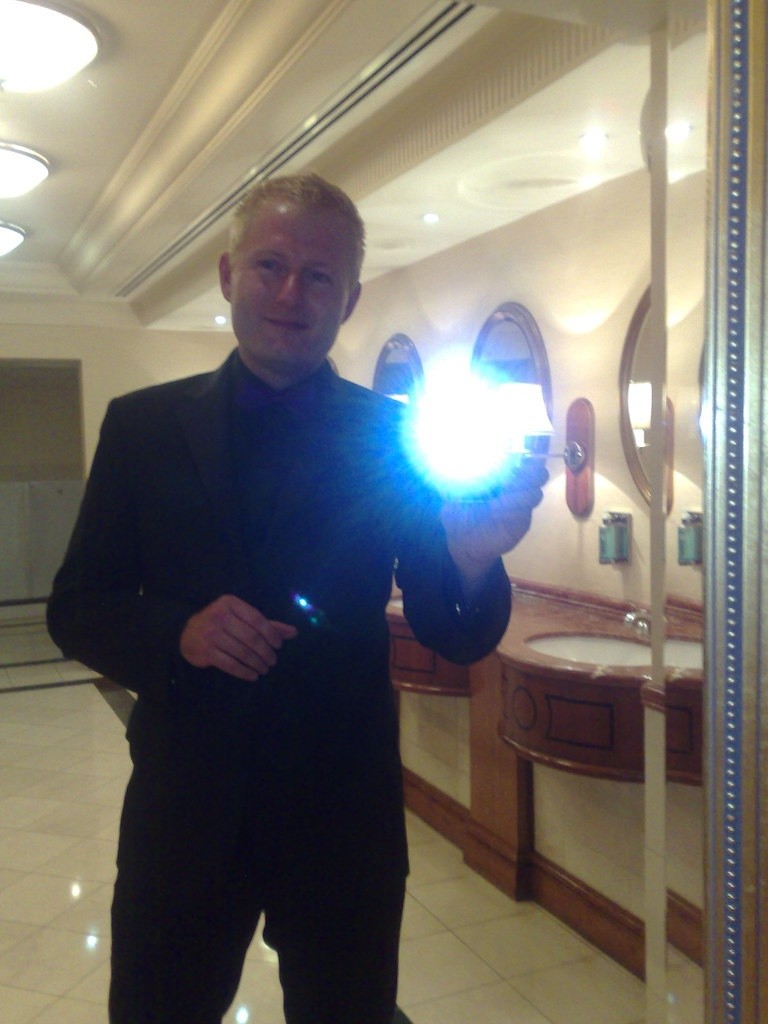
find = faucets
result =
[623,607,653,631]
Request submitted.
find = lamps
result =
[495,379,597,516]
[0,0,123,259]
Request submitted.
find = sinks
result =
[522,633,704,671]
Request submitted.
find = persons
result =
[45,176,550,1024]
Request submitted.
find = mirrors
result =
[618,285,658,506]
[0,0,767,1024]
[474,303,553,429]
[372,332,425,408]
